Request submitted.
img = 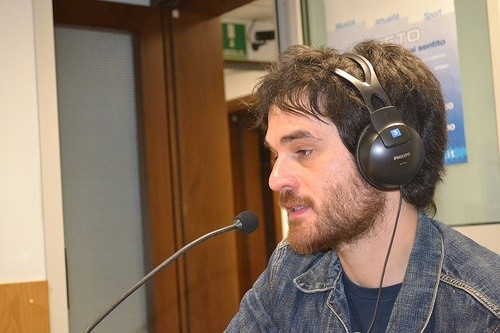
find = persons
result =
[221,38,500,332]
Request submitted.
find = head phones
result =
[318,50,426,191]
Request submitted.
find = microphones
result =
[82,210,259,333]
